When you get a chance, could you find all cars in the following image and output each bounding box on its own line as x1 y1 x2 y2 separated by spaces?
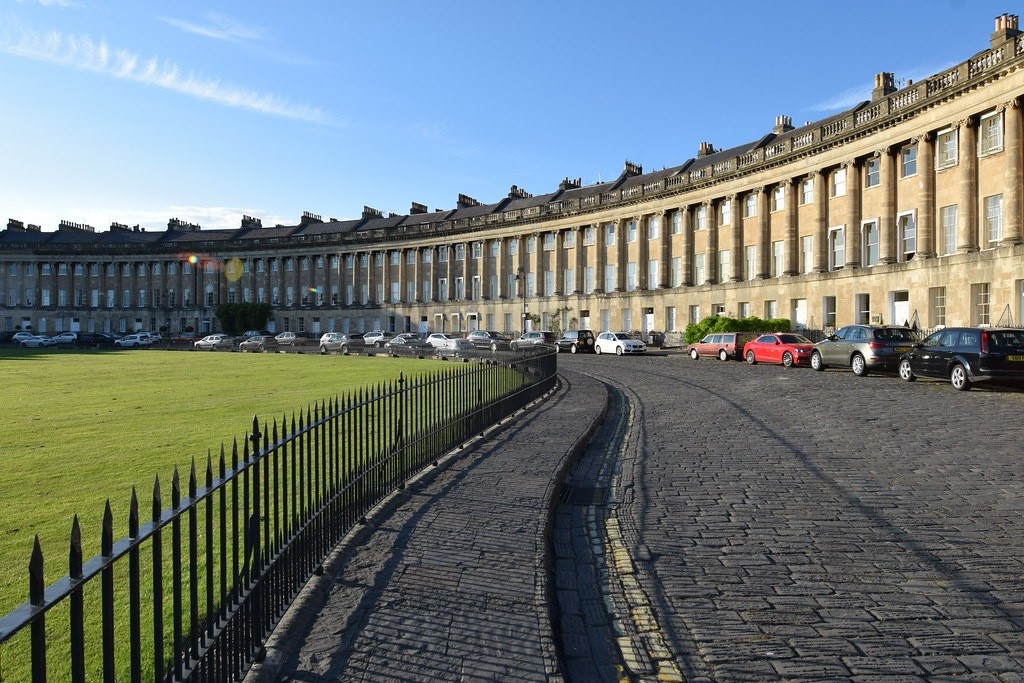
171 333 200 344
319 331 341 344
436 341 478 358
12 332 33 343
239 336 280 353
594 332 647 356
743 333 816 366
20 335 51 347
390 336 434 358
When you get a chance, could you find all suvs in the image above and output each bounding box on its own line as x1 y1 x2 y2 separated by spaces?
235 330 273 345
52 332 77 345
810 323 918 378
114 334 150 348
553 329 595 354
194 334 234 351
426 334 471 349
509 332 556 351
138 332 162 343
384 332 422 349
319 334 365 354
467 331 510 351
274 332 306 346
362 330 393 348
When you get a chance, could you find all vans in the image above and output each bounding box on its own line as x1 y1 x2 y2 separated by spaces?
687 333 758 361
79 332 114 346
897 326 1024 392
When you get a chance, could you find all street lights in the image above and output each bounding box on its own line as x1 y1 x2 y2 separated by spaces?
514 267 526 334
